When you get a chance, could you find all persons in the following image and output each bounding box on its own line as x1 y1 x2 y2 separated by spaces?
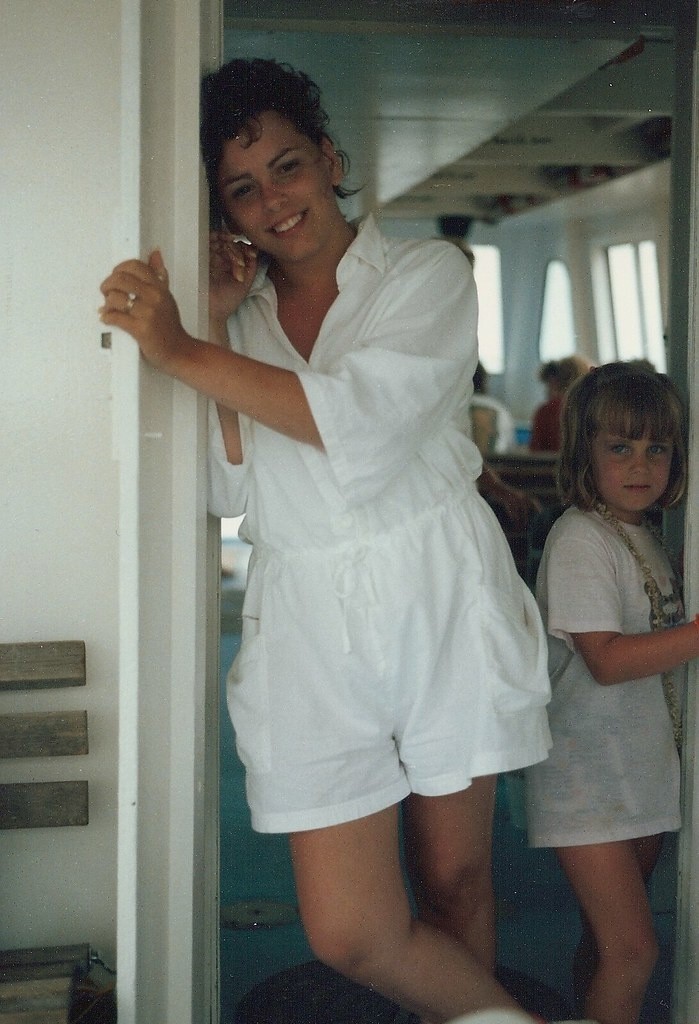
97 59 555 1024
527 360 699 1024
471 353 592 529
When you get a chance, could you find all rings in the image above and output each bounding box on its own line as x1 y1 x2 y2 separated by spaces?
123 293 137 314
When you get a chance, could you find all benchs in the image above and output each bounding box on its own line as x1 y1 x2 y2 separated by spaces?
1 641 89 1023
481 454 664 548
